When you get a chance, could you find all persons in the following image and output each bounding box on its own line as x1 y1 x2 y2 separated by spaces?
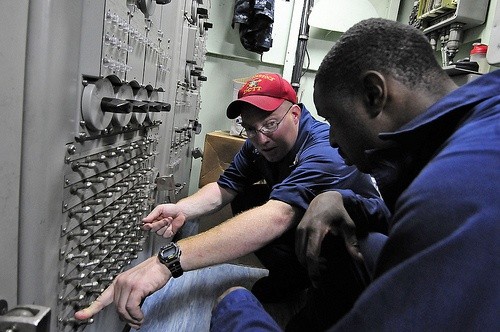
76 71 380 332
208 17 500 332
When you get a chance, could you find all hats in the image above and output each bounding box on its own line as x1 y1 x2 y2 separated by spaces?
226 72 298 119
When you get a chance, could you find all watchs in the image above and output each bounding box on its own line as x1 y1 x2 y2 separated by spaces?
157 241 184 279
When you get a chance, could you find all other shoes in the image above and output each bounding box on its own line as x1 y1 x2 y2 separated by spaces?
285 298 355 332
251 277 310 303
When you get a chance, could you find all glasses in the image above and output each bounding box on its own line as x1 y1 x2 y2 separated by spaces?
238 105 292 139
